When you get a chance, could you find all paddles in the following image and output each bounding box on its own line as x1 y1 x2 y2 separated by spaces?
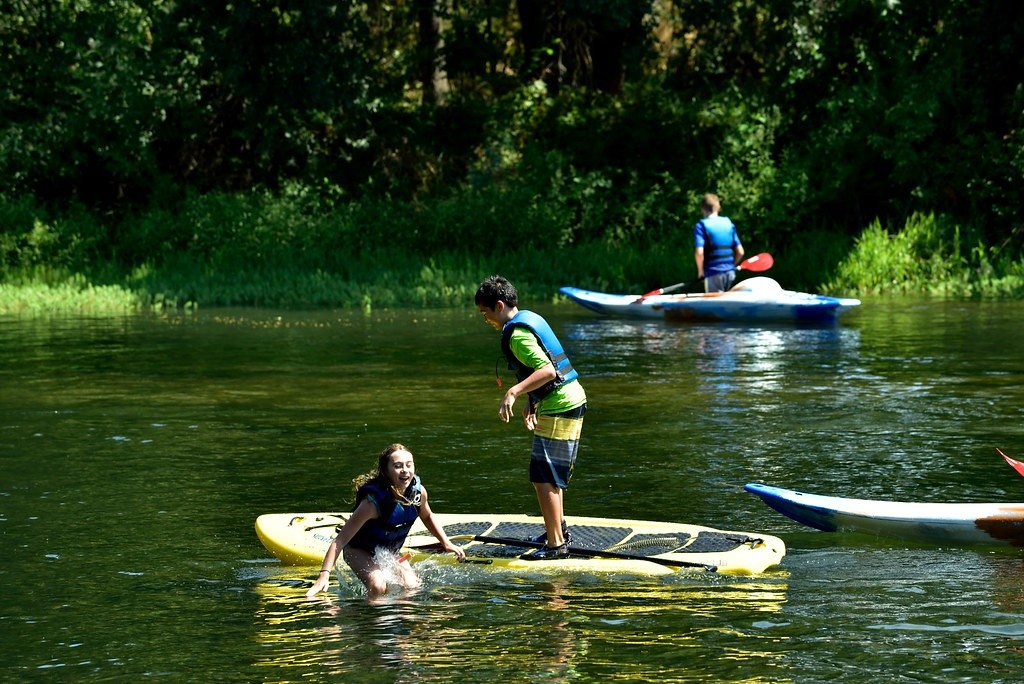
638 252 773 300
402 534 717 573
995 447 1024 477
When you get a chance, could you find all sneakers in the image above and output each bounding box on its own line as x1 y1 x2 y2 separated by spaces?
522 545 572 561
522 530 571 544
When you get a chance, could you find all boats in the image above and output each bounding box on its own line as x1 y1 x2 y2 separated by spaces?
560 276 863 322
744 480 1024 547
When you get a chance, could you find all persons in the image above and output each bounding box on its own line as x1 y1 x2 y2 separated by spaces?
693 192 744 292
306 444 464 597
474 275 588 559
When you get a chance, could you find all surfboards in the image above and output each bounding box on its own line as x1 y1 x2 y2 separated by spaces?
251 508 787 581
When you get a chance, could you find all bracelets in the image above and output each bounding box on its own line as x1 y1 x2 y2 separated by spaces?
320 570 329 573
